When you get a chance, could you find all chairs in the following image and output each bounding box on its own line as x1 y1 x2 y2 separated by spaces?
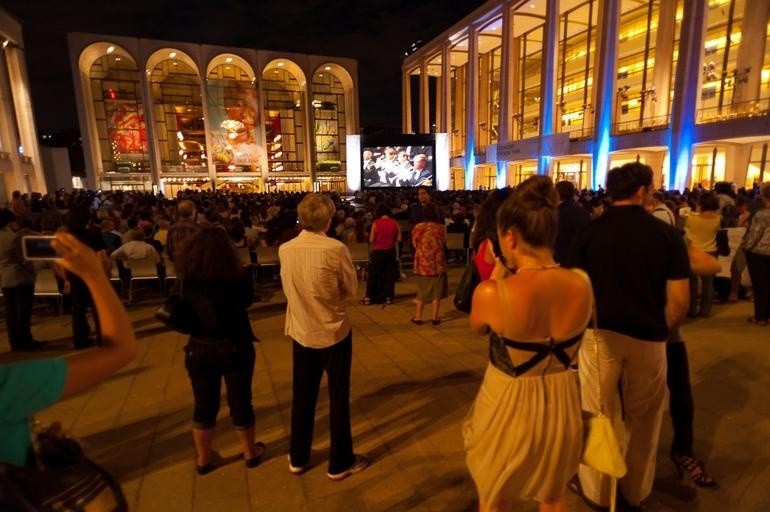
346 242 370 267
33 269 67 327
127 258 162 305
253 246 279 288
103 257 124 300
445 232 466 250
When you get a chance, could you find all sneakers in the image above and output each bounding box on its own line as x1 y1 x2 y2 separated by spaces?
327 454 368 479
196 450 215 475
287 452 304 474
246 441 265 468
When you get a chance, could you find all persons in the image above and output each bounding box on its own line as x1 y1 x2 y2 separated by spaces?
741 183 770 327
0 208 44 353
205 188 299 228
114 188 166 238
676 182 753 210
164 228 266 476
683 193 720 319
722 185 769 304
553 180 591 262
567 169 690 512
278 193 370 481
620 163 715 487
177 189 205 200
30 187 70 233
359 204 401 306
362 146 434 186
70 207 112 350
648 191 675 227
410 203 448 325
70 188 114 228
461 175 595 512
0 233 139 512
574 184 607 211
166 200 206 281
111 229 160 277
332 193 370 245
409 186 440 228
12 191 26 213
471 186 513 281
440 186 489 233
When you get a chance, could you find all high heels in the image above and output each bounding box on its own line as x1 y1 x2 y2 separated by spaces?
670 454 715 487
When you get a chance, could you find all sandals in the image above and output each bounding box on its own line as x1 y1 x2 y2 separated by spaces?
359 297 371 305
386 298 391 304
411 317 422 325
433 317 440 325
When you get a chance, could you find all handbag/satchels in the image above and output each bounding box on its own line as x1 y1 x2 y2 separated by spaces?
0 441 127 512
155 286 191 337
453 263 479 313
573 266 628 479
715 221 731 256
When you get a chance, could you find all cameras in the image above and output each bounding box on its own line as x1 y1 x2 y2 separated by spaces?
19 235 63 261
